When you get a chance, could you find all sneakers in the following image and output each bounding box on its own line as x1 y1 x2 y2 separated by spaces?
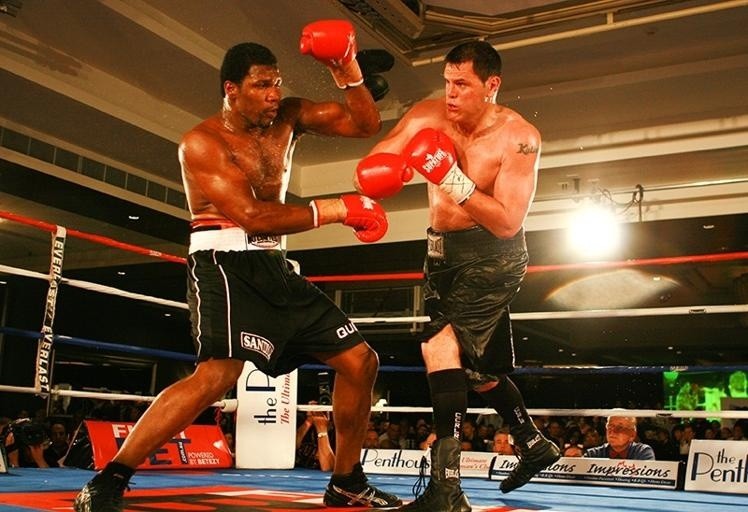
322 461 403 509
74 460 137 512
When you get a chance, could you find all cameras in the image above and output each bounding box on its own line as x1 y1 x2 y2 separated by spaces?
318 372 332 405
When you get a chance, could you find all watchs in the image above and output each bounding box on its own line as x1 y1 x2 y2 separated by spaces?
317 432 327 438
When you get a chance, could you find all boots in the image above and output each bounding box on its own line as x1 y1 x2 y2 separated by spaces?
499 417 563 495
389 434 474 512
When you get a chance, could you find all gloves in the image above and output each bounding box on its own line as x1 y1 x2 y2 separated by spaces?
299 19 365 89
311 152 410 244
405 126 477 206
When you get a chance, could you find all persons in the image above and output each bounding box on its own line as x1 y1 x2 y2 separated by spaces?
354 41 561 512
1 388 152 470
219 416 235 458
534 406 748 460
76 17 405 512
362 402 513 455
676 378 705 410
296 375 336 472
